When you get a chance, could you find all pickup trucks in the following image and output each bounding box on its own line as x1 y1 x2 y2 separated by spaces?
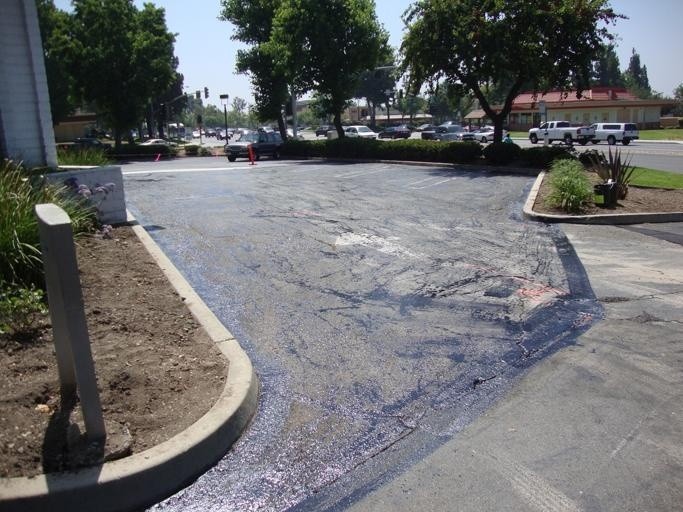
527 119 595 146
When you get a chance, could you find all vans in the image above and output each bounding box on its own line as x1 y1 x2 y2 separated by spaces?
589 122 638 145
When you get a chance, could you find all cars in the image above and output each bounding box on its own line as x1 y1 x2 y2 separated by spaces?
86 128 111 138
135 123 300 162
313 122 511 142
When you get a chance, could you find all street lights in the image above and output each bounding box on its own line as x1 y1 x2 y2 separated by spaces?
218 94 229 143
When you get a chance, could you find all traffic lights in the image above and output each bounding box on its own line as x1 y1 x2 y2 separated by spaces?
203 87 208 99
